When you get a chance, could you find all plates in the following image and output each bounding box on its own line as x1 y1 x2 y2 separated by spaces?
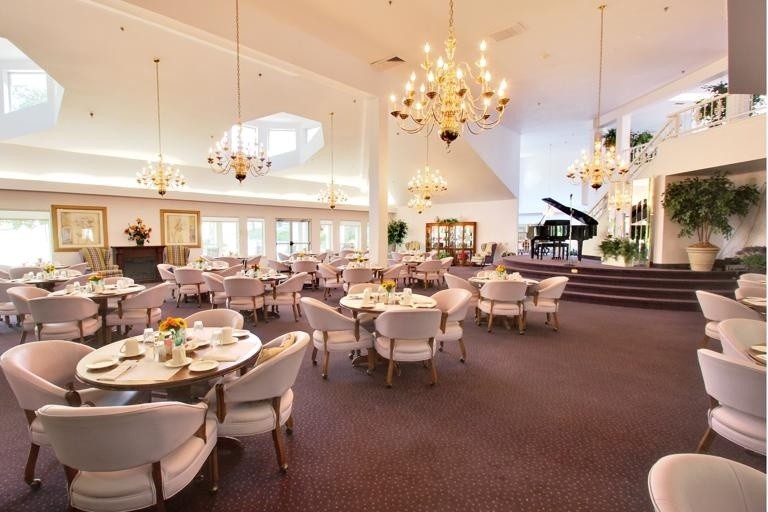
215 337 238 345
188 360 219 371
231 330 249 337
85 356 119 370
118 349 144 358
184 338 209 354
164 356 193 368
751 345 766 352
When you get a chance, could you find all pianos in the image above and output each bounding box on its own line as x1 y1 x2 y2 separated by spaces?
529 197 598 261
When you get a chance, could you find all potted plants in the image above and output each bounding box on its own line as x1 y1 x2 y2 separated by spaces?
655 164 763 274
596 231 643 270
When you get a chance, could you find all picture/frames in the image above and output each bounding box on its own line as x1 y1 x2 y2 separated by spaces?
158 207 202 249
49 203 110 253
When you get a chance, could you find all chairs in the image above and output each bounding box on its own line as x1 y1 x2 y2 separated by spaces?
632 262 768 510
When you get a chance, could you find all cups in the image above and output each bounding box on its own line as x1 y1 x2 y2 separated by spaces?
236 269 276 278
23 269 70 281
361 286 414 308
187 263 208 270
210 336 223 354
120 339 139 356
193 321 204 336
172 345 187 364
65 279 138 295
220 327 233 341
509 272 520 280
156 335 172 362
143 328 154 346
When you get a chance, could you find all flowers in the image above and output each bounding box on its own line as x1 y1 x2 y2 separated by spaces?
123 216 154 244
